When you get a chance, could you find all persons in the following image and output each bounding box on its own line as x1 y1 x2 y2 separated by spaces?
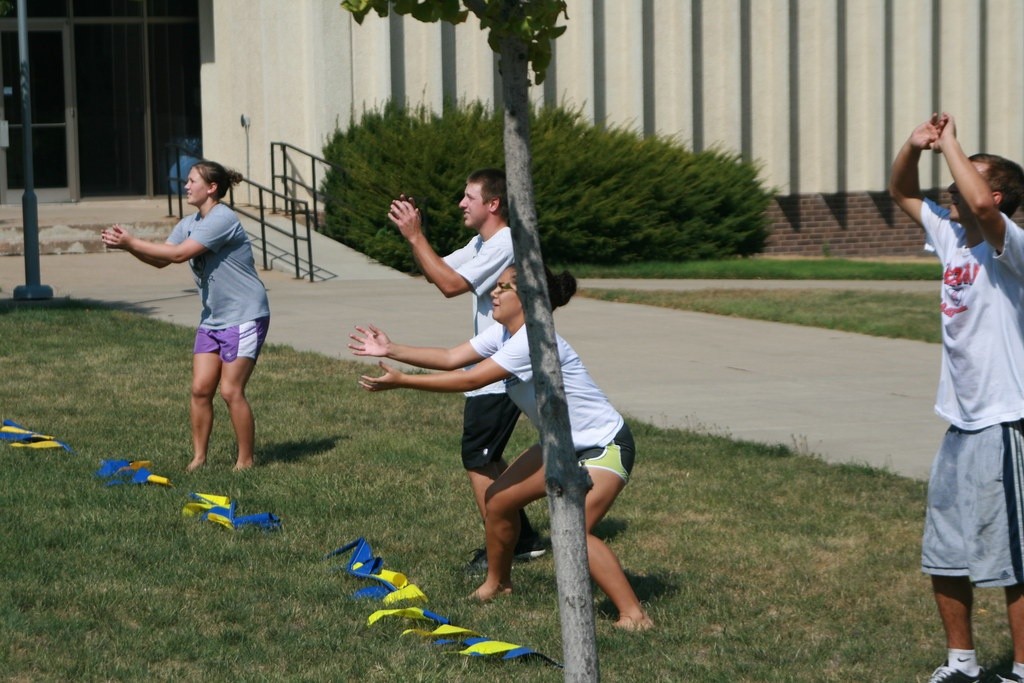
386 169 549 580
347 262 656 635
888 111 1023 683
103 163 273 477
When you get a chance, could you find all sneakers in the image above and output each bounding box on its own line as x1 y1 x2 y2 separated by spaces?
926 659 985 683
989 671 1024 683
513 533 546 558
470 547 488 576
